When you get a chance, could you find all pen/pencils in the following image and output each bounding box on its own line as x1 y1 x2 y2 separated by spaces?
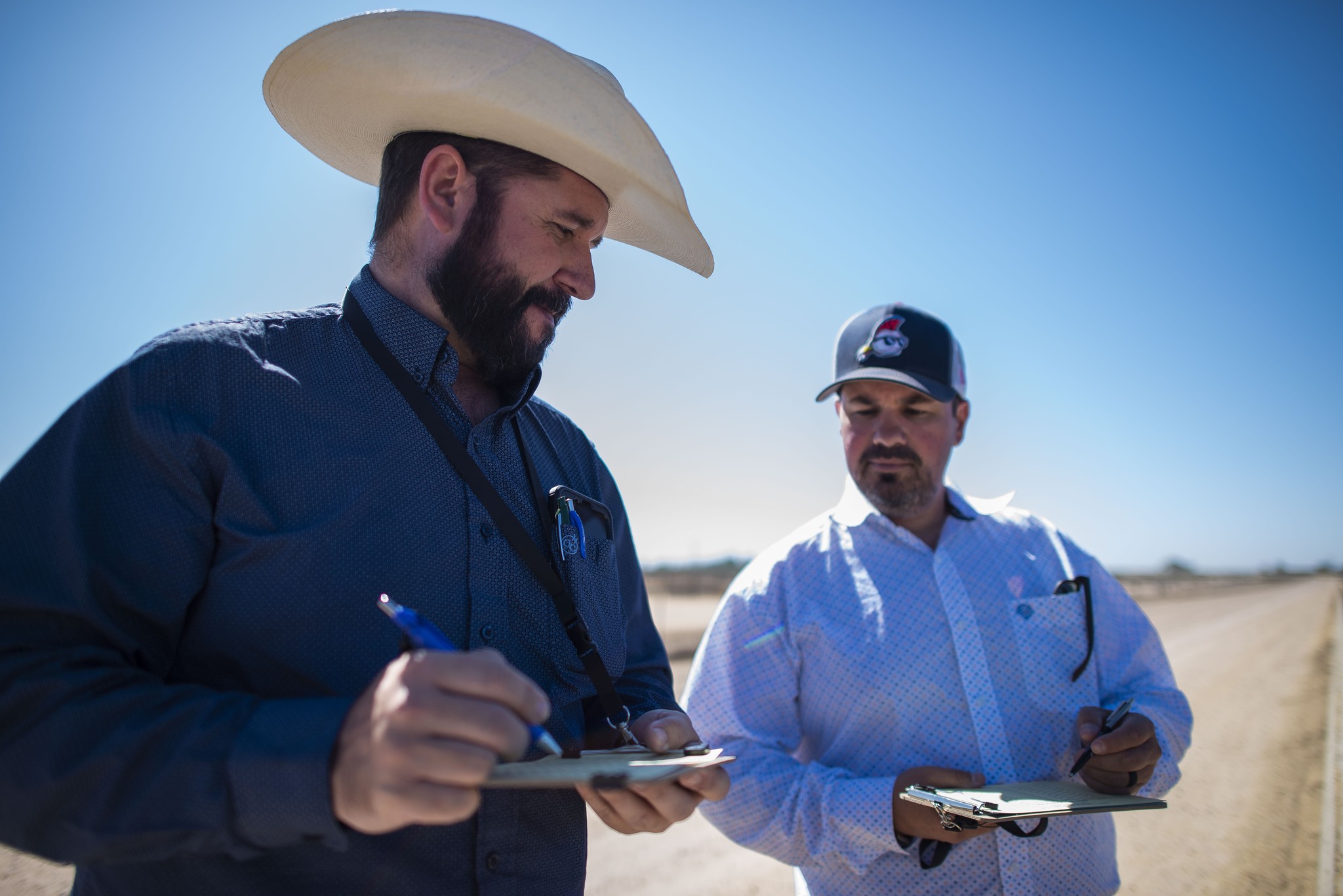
566 498 589 558
1068 695 1133 781
376 594 573 757
555 497 571 560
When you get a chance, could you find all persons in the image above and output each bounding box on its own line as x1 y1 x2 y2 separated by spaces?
676 301 1193 895
0 9 728 896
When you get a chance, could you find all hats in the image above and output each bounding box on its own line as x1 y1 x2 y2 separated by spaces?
261 10 719 282
816 303 970 408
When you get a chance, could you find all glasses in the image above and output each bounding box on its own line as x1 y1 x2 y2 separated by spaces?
1053 576 1095 681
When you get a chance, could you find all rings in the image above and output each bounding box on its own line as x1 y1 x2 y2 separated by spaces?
1126 771 1137 787
